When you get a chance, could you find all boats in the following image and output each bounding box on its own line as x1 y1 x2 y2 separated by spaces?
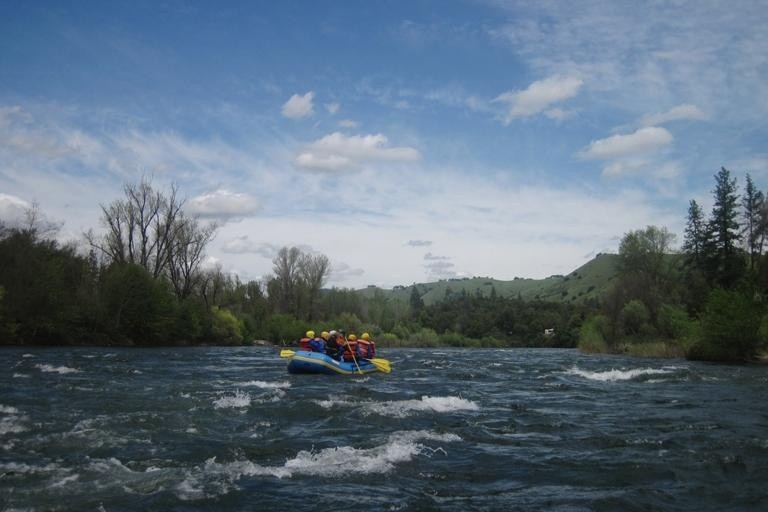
287 350 381 376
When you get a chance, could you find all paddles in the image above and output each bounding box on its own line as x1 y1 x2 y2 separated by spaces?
363 358 390 372
280 349 297 357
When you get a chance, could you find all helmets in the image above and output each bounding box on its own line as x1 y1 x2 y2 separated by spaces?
305 329 369 341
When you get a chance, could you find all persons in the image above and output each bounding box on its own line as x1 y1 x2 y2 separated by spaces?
300 329 376 362
